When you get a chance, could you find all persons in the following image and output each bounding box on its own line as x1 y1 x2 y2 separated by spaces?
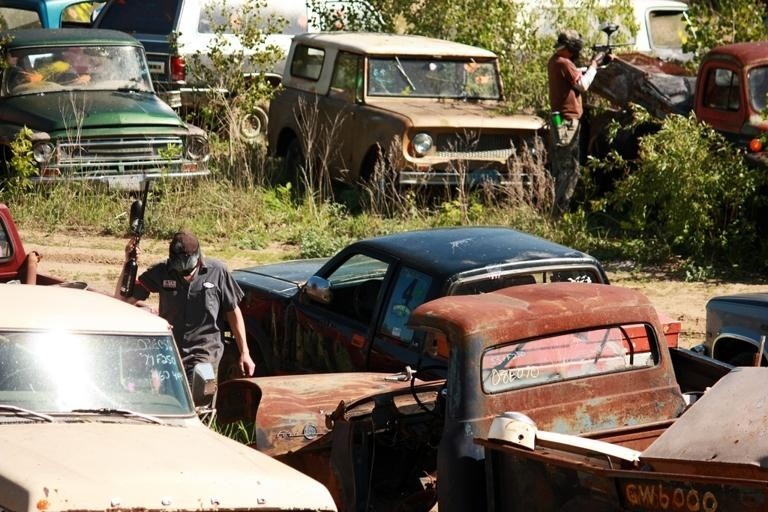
547 30 603 211
113 229 255 424
25 248 43 286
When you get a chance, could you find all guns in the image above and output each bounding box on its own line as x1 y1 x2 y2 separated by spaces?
120 181 149 296
589 25 634 65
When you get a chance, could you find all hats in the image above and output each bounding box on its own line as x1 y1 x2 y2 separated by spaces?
553 27 580 49
168 229 201 276
